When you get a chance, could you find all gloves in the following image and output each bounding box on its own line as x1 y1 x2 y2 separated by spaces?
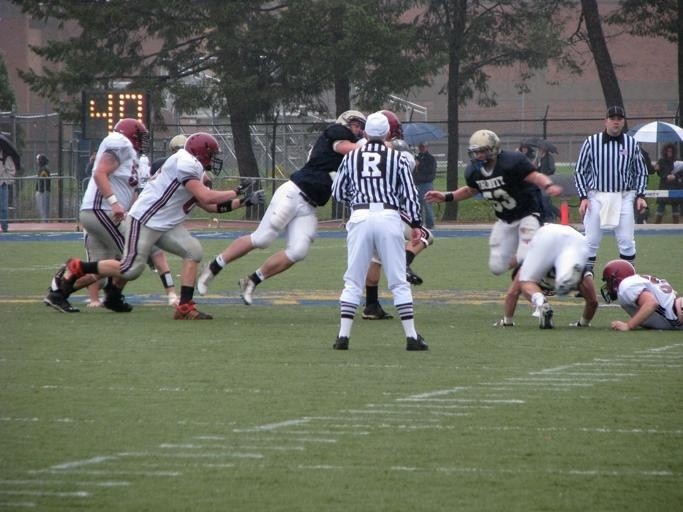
569 315 592 327
234 178 257 195
494 319 515 328
240 189 266 206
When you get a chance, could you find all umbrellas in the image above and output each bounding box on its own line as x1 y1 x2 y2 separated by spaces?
627 118 683 160
1 135 21 170
520 135 559 161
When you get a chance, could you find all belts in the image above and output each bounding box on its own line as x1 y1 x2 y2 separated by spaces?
299 192 318 208
353 202 398 211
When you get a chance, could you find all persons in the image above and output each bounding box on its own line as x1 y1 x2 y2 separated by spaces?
635 141 658 223
1 147 15 233
494 223 598 328
602 259 682 330
656 144 683 223
196 109 435 351
43 118 267 319
34 154 50 223
573 106 650 298
516 139 535 159
423 129 563 280
537 149 560 224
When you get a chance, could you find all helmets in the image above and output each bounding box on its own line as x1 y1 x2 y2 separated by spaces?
539 267 556 295
336 110 399 136
113 118 152 155
36 154 49 162
467 129 502 169
601 260 635 304
170 132 223 177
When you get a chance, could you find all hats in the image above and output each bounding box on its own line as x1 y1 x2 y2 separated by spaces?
607 107 626 118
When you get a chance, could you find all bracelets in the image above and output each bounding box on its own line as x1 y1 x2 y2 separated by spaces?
445 191 454 202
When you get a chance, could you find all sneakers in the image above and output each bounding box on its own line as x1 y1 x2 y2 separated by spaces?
406 264 423 285
406 333 428 350
102 277 133 312
539 303 554 328
333 336 350 350
45 290 80 313
174 300 213 319
362 302 395 320
239 275 256 306
52 259 85 292
197 261 215 295
